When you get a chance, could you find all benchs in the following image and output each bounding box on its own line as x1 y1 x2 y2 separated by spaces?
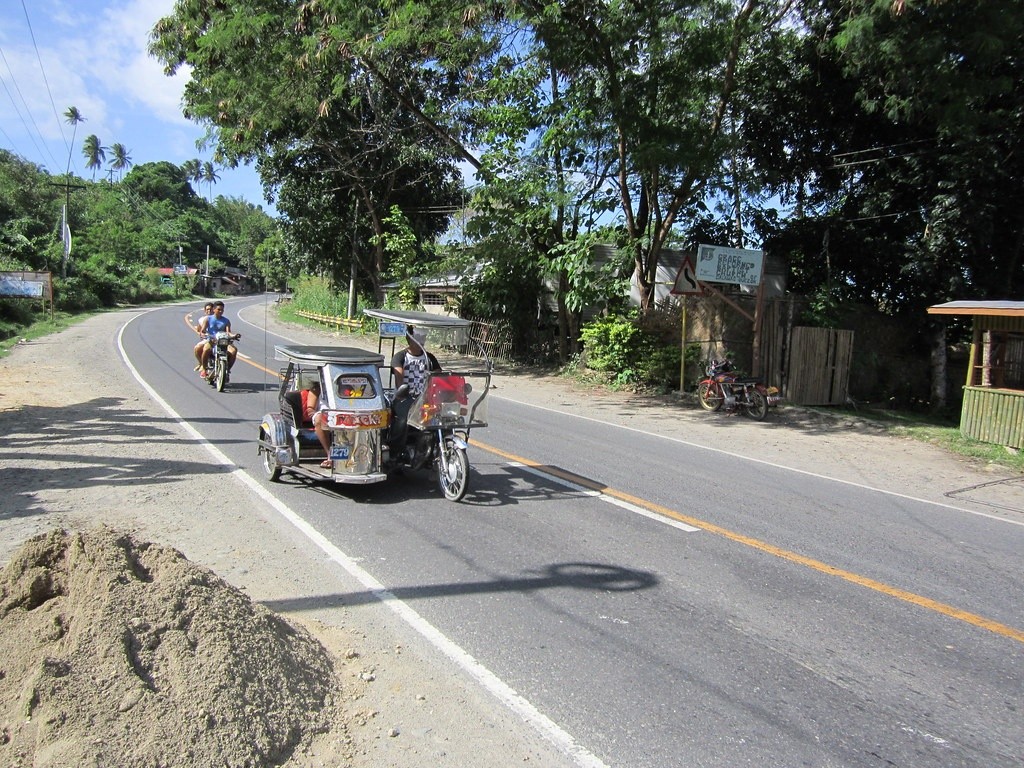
286 392 322 447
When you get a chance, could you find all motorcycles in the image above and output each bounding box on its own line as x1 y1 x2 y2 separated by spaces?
201 328 241 393
697 361 780 422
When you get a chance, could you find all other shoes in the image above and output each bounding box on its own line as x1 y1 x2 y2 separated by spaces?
194 365 201 371
321 461 331 468
200 371 206 378
388 458 403 465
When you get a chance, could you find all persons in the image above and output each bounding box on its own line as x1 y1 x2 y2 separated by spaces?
307 381 338 468
389 325 446 466
194 301 241 384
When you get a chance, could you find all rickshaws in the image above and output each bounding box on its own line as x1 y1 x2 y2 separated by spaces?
257 308 499 504
